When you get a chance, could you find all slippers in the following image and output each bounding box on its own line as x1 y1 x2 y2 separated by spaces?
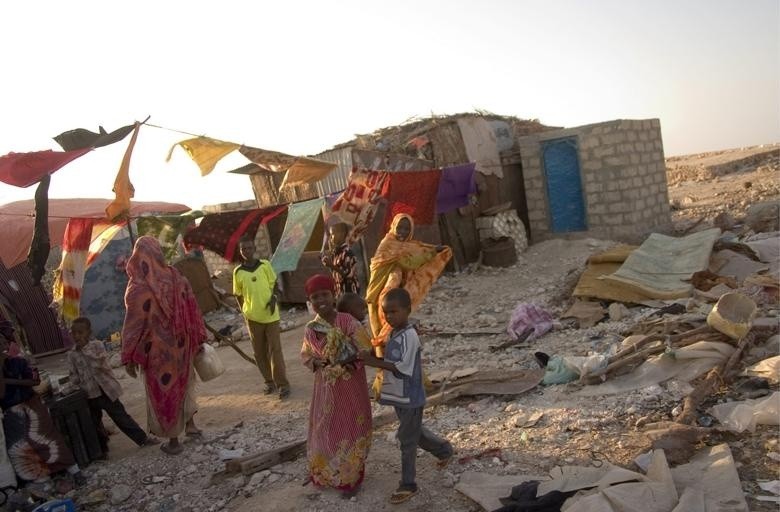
390 485 420 504
436 457 452 472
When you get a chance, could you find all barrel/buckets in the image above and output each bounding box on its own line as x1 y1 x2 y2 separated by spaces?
195 342 224 382
110 332 121 342
479 237 518 266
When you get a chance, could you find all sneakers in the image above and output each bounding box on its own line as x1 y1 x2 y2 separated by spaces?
185 428 202 437
145 435 161 445
72 471 98 490
160 442 183 454
278 387 290 399
264 385 275 396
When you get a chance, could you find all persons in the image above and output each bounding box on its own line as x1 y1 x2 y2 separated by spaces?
355 288 453 505
0 320 26 357
232 236 291 400
0 333 82 496
364 213 454 400
299 272 373 498
67 317 163 453
119 236 208 455
321 222 360 302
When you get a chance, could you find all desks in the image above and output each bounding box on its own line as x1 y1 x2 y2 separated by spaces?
42 387 103 466
